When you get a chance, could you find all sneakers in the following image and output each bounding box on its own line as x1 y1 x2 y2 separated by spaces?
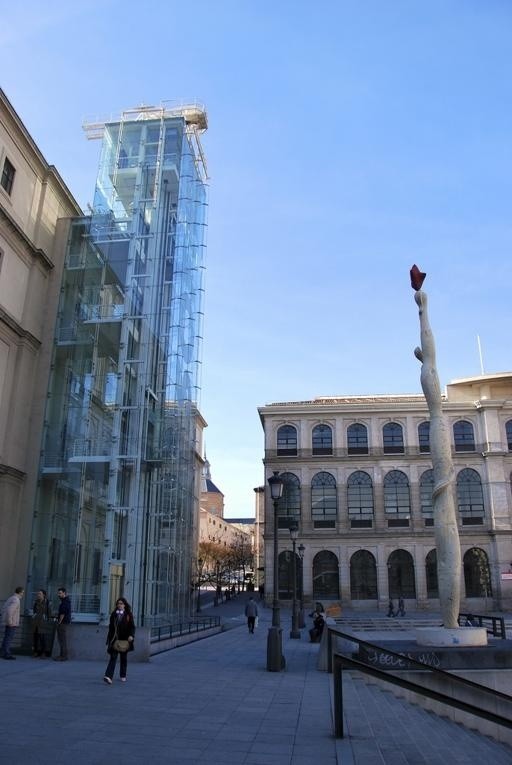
104 677 112 684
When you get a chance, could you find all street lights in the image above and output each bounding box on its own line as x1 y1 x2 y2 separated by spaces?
267 471 287 672
227 559 231 600
297 544 305 628
215 560 221 606
196 557 204 613
288 520 301 639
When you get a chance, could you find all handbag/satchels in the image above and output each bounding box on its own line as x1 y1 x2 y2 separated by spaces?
114 640 129 652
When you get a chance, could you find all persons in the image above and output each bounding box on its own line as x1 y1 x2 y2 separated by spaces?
31 590 50 658
224 589 229 601
232 585 235 599
309 602 324 643
386 596 409 619
245 596 259 634
1 587 25 660
104 598 135 684
54 588 72 661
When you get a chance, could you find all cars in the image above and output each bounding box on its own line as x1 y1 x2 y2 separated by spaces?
200 575 209 582
207 571 215 577
211 566 244 585
